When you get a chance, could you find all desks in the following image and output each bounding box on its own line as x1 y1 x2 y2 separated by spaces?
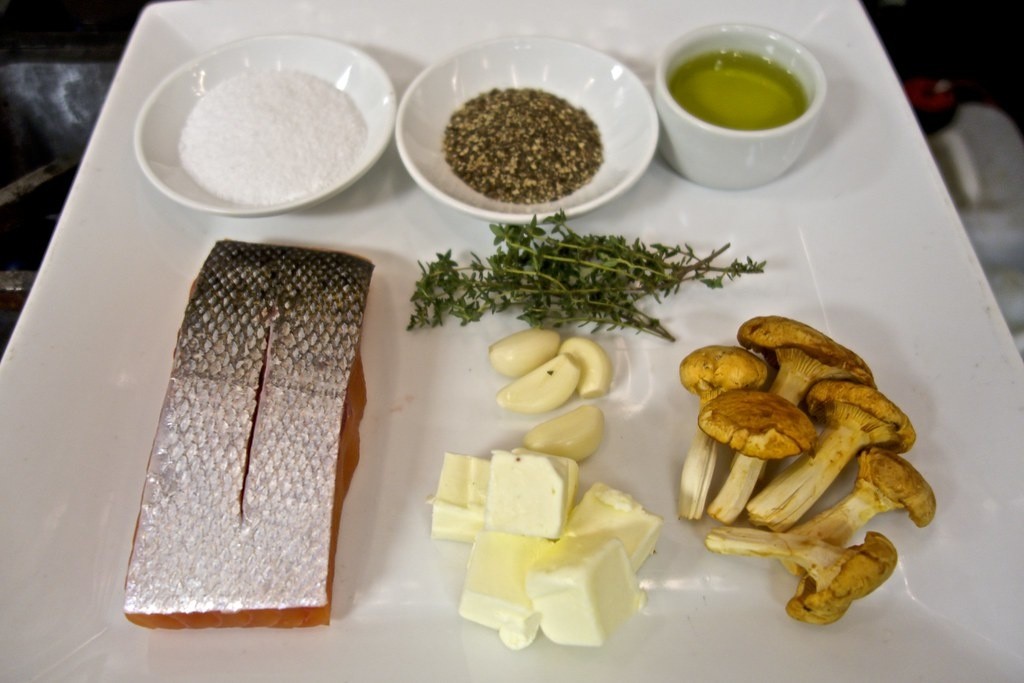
0 0 1024 683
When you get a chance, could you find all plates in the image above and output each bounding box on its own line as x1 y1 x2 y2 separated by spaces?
395 36 661 224
0 0 1024 683
133 32 398 218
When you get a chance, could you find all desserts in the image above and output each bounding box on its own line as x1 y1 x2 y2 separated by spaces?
426 445 662 648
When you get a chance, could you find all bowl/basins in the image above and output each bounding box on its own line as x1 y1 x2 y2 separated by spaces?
651 23 829 194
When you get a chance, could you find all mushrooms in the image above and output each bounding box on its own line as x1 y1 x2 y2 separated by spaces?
677 315 936 625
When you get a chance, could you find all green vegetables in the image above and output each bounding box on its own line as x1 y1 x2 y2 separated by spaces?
405 208 767 342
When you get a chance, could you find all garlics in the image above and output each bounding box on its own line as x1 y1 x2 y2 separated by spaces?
487 328 611 463
